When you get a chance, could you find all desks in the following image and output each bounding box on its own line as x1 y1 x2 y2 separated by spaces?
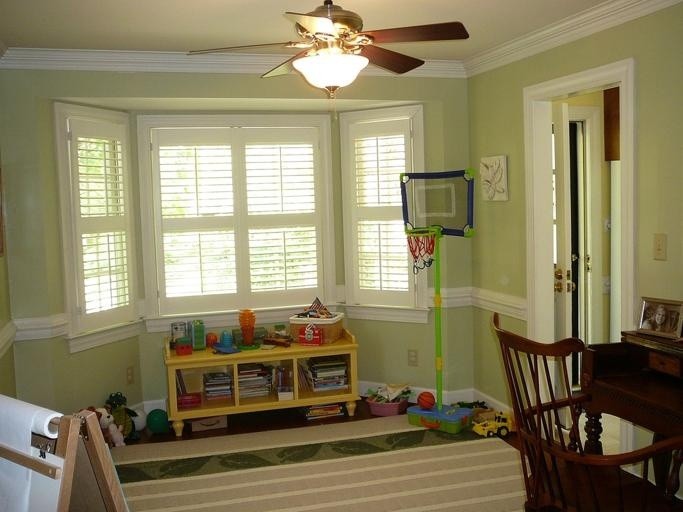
581 326 682 493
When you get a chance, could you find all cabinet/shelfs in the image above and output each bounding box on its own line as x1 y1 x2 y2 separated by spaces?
160 330 361 437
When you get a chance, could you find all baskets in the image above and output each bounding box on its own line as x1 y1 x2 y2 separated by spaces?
366 395 408 416
289 312 344 344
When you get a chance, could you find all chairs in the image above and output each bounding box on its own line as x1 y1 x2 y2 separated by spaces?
492 312 682 512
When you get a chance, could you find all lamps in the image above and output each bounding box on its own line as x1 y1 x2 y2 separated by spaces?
292 49 368 96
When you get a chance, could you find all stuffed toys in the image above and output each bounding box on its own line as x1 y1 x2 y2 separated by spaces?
88 392 172 446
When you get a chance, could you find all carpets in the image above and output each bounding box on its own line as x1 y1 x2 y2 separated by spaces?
109 412 534 512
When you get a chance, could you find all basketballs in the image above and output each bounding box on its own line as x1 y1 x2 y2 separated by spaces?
417 392 435 409
206 333 217 346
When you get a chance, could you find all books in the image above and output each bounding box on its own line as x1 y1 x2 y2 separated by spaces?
173 356 350 420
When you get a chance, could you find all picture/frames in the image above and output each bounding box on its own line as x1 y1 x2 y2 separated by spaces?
635 296 682 340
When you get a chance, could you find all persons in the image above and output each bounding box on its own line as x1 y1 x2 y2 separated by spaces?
640 303 671 330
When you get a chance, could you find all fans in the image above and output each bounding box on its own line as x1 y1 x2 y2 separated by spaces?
187 0 468 79
